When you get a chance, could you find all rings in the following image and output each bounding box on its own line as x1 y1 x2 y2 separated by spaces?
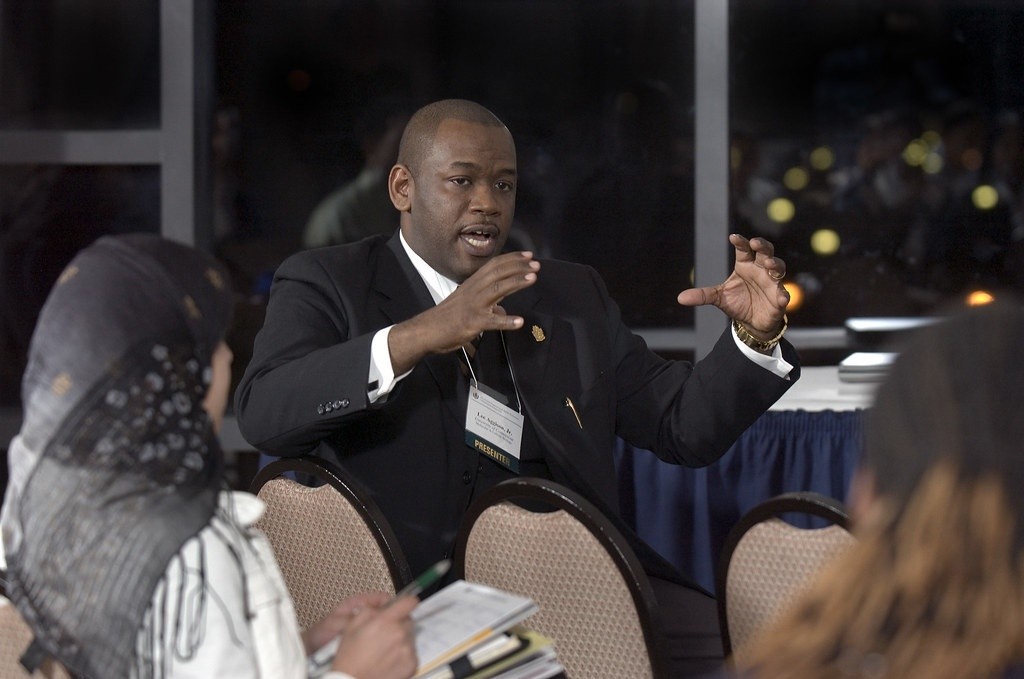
768 270 786 280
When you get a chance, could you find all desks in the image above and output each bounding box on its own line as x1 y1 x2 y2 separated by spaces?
603 367 888 595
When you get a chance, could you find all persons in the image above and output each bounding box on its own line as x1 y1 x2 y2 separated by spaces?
726 300 1024 679
0 235 421 679
235 100 801 679
301 114 418 251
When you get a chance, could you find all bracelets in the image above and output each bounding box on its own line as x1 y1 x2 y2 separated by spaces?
733 313 789 351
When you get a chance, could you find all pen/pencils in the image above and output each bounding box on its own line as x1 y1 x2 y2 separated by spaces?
377 558 452 613
562 396 583 431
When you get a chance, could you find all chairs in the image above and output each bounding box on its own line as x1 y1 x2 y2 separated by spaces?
452 478 667 679
718 492 859 679
249 454 413 633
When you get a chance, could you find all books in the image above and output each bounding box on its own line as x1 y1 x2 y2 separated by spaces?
415 625 565 679
